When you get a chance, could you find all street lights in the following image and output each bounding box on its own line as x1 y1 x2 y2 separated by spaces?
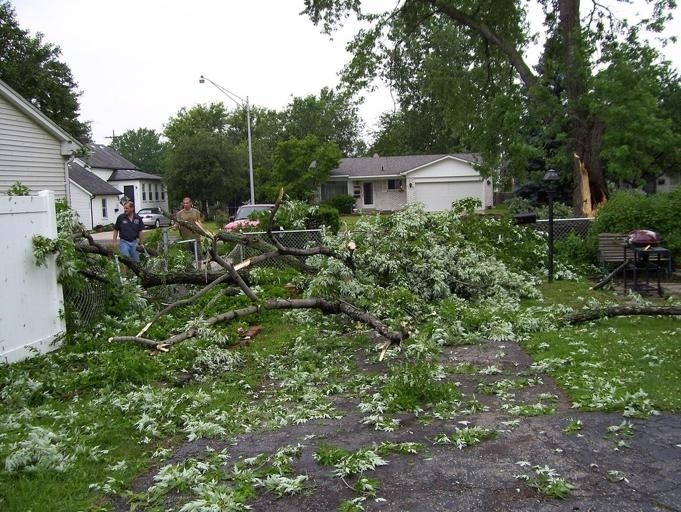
542 166 564 284
198 73 257 204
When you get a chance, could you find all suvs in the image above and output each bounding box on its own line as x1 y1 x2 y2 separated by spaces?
223 201 282 242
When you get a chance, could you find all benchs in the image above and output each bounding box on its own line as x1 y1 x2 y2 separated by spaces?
596 231 670 281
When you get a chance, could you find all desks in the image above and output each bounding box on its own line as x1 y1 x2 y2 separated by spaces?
614 238 669 300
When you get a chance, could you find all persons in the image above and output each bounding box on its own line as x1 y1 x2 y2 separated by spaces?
170 197 202 267
111 201 145 276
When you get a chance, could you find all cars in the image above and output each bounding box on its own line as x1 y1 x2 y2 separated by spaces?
136 206 176 228
